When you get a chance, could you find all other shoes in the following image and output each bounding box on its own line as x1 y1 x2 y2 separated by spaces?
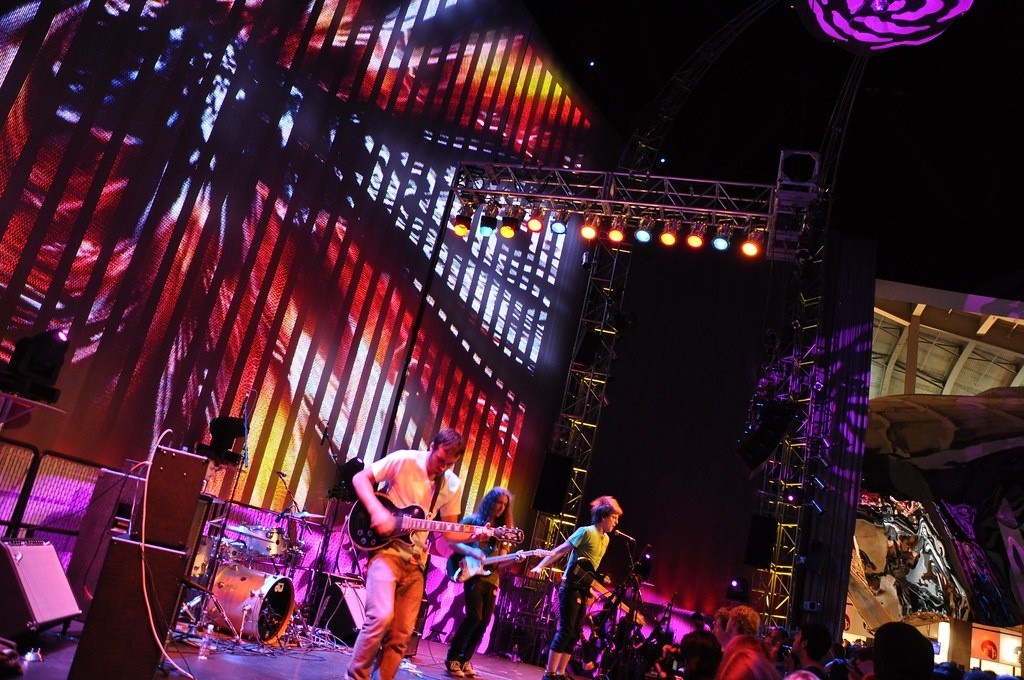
447 661 464 676
541 674 567 680
461 661 476 675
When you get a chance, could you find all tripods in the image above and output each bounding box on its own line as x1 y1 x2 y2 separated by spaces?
178 403 248 647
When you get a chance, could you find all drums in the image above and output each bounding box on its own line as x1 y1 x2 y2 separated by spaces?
246 526 286 558
190 535 215 578
201 561 295 645
210 537 248 562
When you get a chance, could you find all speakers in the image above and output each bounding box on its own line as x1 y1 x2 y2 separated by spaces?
530 452 577 514
804 539 832 575
737 399 798 471
67 468 145 621
129 445 210 548
309 581 369 644
68 536 188 680
0 537 82 645
743 512 778 573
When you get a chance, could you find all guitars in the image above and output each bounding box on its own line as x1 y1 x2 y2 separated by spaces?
566 557 647 627
446 546 556 583
348 492 525 551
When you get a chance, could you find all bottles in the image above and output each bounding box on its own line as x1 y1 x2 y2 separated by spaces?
198 624 214 659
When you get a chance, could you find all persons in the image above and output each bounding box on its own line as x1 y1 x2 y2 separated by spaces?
444 487 526 677
344 430 494 680
656 606 1024 680
530 496 622 680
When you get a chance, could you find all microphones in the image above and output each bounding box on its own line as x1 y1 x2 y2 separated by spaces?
275 509 287 523
321 422 329 445
274 470 286 477
239 393 249 417
615 530 635 543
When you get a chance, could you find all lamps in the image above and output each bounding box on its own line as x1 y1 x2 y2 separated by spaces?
501 205 526 238
550 210 571 234
526 208 546 233
684 221 707 249
739 231 765 256
607 216 627 242
634 215 656 243
479 202 499 237
713 224 733 250
581 214 601 239
451 197 473 237
659 218 682 246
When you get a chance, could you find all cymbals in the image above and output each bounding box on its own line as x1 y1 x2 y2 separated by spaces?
284 512 327 518
207 522 275 543
201 492 225 503
